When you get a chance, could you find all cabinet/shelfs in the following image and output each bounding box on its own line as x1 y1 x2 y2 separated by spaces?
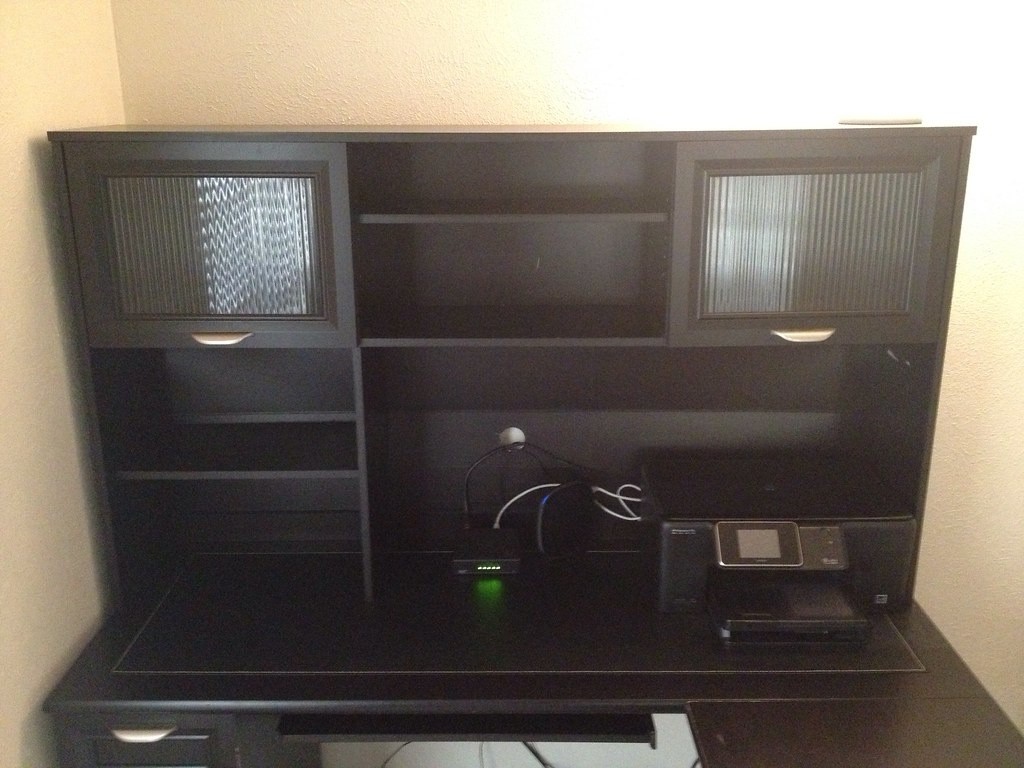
41 119 1022 767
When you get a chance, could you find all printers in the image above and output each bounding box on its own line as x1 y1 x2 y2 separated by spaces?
638 446 917 643
